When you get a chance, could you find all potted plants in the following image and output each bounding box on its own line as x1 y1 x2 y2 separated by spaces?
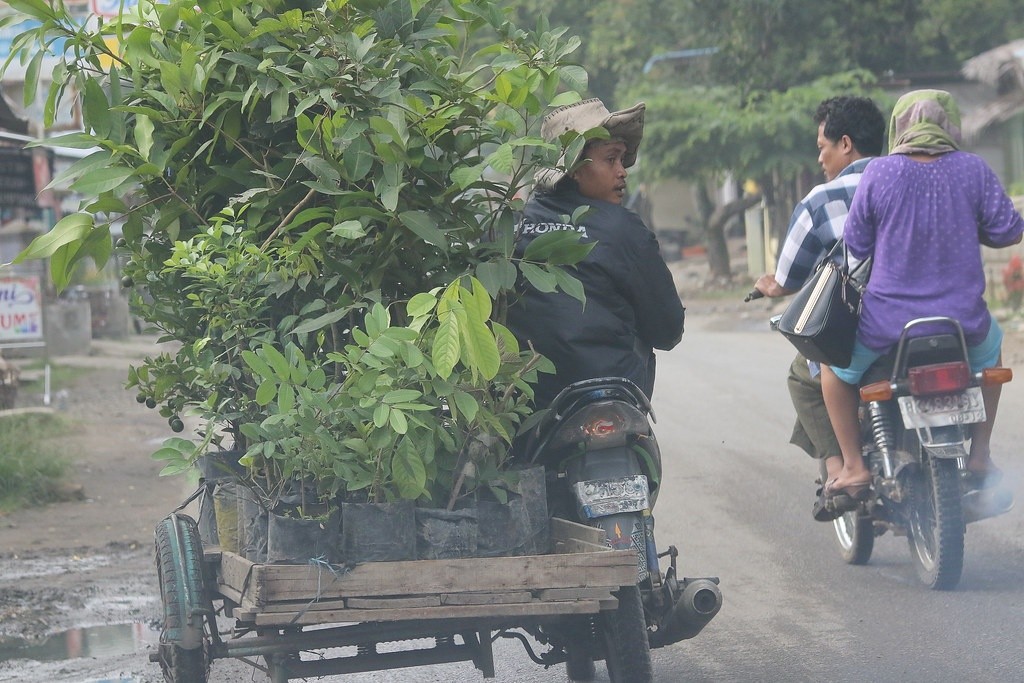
0 1 615 562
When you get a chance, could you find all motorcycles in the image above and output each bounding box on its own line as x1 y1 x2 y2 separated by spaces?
742 269 1017 593
150 352 724 683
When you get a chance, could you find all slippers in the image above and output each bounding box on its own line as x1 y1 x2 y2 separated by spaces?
968 468 1004 489
824 477 872 496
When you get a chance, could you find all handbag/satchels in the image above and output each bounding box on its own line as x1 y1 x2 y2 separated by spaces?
778 235 874 369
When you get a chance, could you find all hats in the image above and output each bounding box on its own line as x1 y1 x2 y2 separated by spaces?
533 96 646 194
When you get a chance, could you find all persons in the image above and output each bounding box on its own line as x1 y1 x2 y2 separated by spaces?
754 95 886 522
823 89 1024 494
471 95 685 461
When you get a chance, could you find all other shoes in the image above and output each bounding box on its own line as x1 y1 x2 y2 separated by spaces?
812 486 845 522
